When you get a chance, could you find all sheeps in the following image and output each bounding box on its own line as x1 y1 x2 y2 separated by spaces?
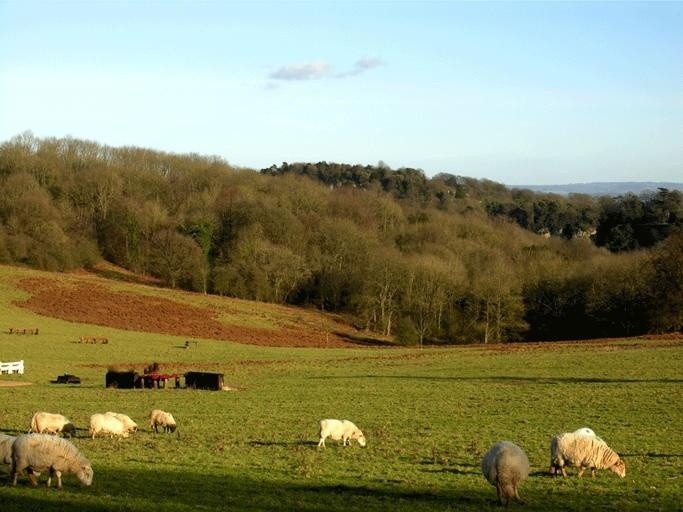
315 418 367 449
481 441 530 505
89 411 138 440
550 426 627 481
28 411 77 438
0 432 93 489
149 409 177 435
